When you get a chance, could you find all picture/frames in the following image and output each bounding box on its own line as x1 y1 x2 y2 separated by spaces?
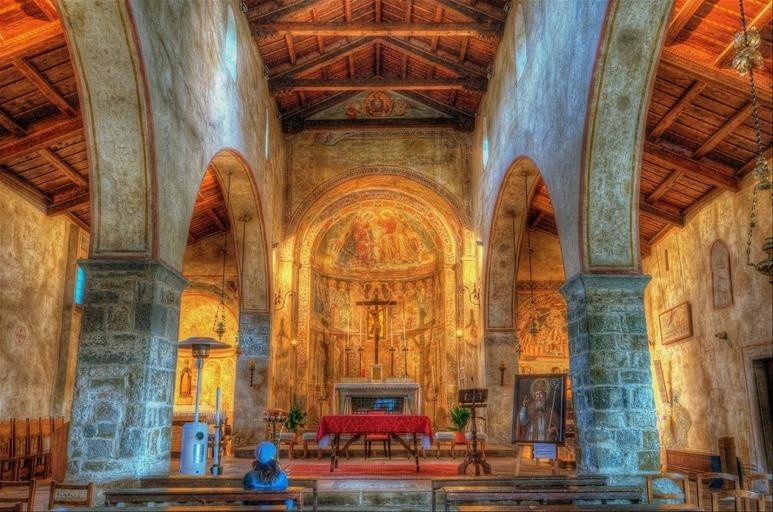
511 373 566 443
371 364 383 384
659 300 693 344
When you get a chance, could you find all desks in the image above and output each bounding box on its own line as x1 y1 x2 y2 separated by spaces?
317 414 431 472
431 475 700 511
435 431 488 459
50 473 319 512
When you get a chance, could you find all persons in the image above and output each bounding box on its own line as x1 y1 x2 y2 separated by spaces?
334 210 428 266
367 304 384 334
516 376 559 441
243 441 295 511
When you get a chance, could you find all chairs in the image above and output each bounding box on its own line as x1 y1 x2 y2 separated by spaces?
262 408 334 459
0 478 94 512
365 410 392 458
644 470 773 512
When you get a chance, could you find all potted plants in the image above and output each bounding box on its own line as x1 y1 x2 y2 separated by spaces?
283 400 308 445
448 406 471 442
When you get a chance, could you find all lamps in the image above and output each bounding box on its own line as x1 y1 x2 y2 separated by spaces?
520 169 542 334
174 336 232 474
247 359 258 387
728 0 773 284
212 217 232 339
